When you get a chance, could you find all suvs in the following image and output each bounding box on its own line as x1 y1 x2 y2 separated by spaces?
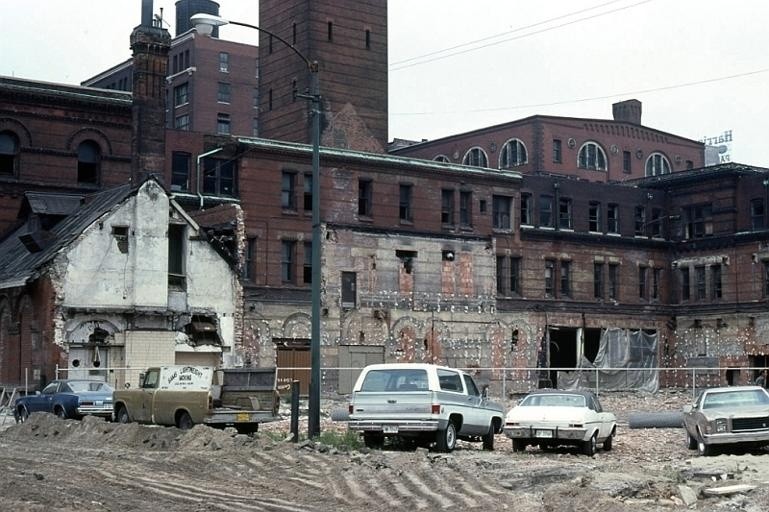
346 361 506 451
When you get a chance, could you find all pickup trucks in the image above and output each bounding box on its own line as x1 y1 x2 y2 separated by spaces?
111 363 282 434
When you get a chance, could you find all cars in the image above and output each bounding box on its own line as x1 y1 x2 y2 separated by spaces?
681 384 769 455
502 388 618 458
13 377 117 423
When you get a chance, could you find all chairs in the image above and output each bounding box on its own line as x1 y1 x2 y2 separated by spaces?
399 384 418 391
440 382 457 391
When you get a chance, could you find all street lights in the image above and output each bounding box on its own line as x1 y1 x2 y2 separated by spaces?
188 12 325 439
